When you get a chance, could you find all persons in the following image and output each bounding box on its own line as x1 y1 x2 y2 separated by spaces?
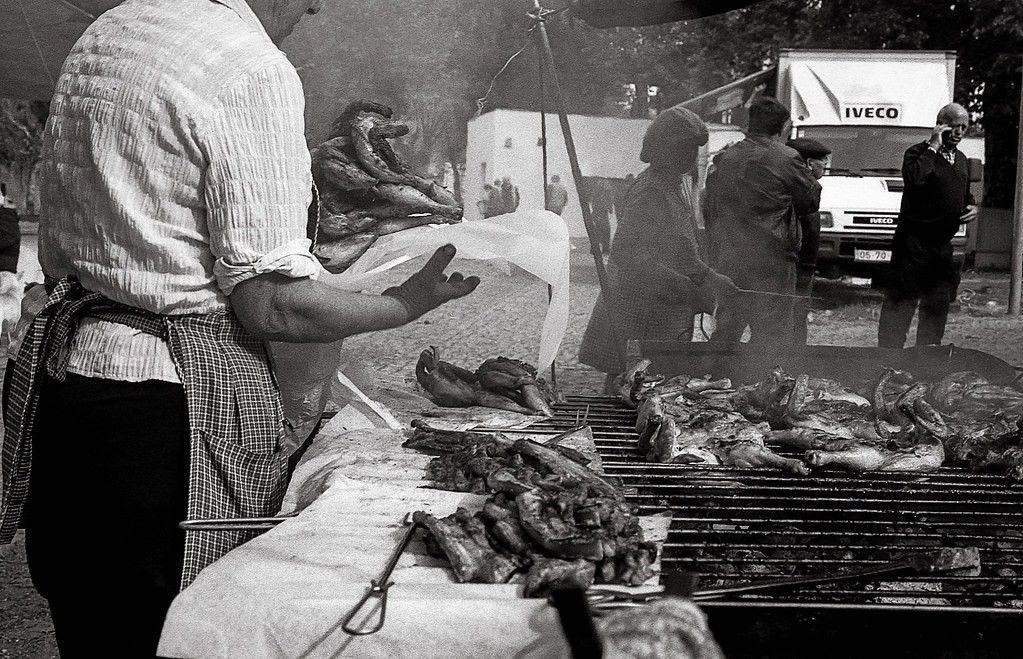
0 0 480 658
476 178 520 219
546 175 568 216
0 181 22 272
590 174 639 254
878 102 980 351
577 106 740 396
699 97 833 346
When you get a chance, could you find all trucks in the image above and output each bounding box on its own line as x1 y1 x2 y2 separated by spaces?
660 51 983 267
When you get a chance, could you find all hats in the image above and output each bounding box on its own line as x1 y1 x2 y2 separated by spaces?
640 106 709 162
786 138 831 157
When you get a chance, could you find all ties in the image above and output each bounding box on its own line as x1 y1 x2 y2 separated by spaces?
948 150 955 165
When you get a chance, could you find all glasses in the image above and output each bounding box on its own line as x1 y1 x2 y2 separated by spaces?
817 158 829 166
